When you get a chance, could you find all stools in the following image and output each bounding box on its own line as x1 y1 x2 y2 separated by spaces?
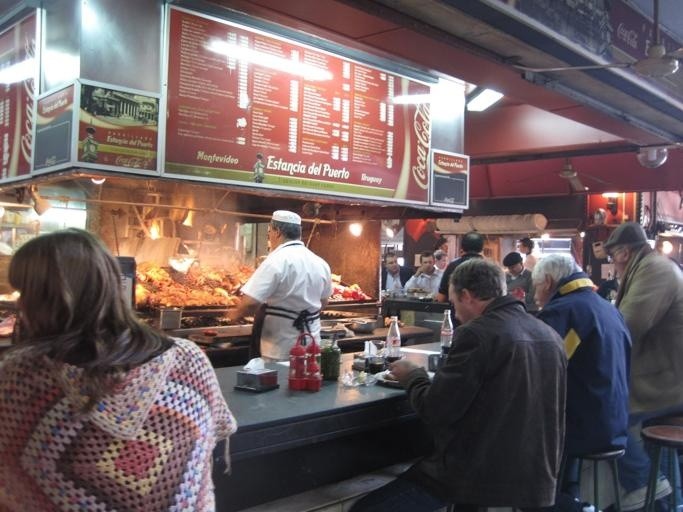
641 414 683 512
574 445 626 512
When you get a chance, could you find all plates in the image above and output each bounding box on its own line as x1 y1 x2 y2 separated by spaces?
374 370 436 385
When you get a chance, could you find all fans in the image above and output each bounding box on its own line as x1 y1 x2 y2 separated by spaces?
510 1 683 80
517 157 611 192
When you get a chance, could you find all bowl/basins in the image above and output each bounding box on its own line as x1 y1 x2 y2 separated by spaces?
349 318 377 333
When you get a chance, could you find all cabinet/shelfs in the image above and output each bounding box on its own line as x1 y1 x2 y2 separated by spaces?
0 202 40 256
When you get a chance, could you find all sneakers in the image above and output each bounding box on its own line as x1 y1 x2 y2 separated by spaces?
614 475 674 512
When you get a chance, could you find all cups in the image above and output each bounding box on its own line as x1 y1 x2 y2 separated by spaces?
428 354 449 371
367 340 386 366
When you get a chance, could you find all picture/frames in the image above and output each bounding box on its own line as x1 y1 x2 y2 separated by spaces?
32 80 73 176
73 77 163 177
159 3 434 206
0 7 41 185
431 149 470 209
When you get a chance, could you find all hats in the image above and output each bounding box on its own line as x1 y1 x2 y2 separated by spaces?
272 210 301 225
603 221 647 247
503 253 522 266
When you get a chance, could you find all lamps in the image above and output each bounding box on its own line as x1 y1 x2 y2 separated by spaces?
29 184 51 216
349 218 368 237
466 87 504 113
636 145 669 169
182 211 193 228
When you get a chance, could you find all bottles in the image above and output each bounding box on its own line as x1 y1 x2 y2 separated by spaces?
440 308 454 354
288 333 341 393
386 315 401 357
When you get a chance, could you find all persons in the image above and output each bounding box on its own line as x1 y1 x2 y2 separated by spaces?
602 220 683 511
351 259 568 508
224 208 333 362
380 231 540 311
581 224 619 299
0 227 239 512
533 252 633 512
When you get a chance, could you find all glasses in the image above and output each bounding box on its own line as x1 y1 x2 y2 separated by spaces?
608 247 625 258
